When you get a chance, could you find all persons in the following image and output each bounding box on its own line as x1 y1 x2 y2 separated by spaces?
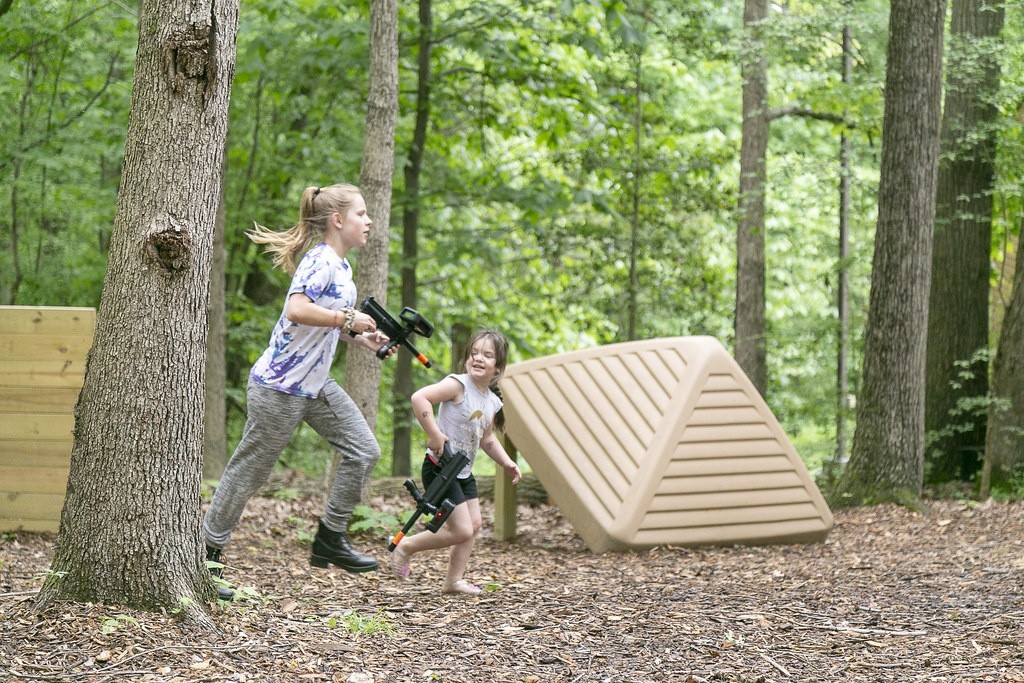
205 183 397 599
393 329 523 597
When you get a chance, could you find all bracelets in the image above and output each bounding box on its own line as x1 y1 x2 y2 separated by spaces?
332 308 338 329
340 305 356 335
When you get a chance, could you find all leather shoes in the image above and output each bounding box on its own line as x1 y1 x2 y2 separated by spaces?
202 544 234 601
308 518 380 570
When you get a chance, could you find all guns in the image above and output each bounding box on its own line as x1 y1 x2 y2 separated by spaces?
388 440 471 553
348 296 433 369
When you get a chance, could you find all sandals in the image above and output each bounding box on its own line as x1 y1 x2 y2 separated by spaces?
387 534 409 581
439 579 482 595
